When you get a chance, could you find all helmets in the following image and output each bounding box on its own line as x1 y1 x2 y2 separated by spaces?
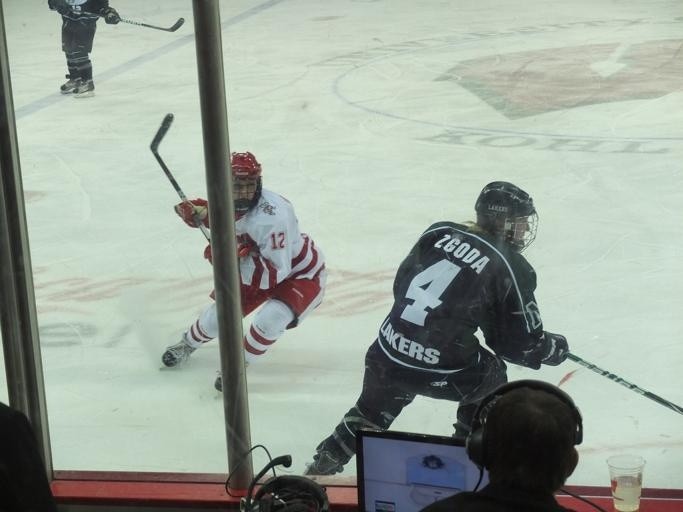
231 152 262 212
475 181 539 253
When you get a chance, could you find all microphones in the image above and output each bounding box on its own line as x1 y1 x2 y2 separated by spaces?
245 455 291 512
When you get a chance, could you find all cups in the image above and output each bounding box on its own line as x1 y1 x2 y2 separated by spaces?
605 452 648 512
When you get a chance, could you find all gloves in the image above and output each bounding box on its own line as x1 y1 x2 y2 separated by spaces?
175 199 208 227
104 8 120 24
543 332 568 366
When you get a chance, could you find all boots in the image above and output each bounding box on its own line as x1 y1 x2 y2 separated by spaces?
163 340 195 367
61 79 94 93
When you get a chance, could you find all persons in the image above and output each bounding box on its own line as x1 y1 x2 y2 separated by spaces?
307 179 570 479
419 385 580 512
48 0 121 95
159 149 329 391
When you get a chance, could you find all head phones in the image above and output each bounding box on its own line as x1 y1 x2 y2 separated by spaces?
466 379 582 472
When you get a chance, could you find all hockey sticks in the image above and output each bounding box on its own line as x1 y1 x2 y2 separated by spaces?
95 12 184 32
149 114 210 241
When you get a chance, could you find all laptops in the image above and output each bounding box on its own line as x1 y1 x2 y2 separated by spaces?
357 428 491 512
